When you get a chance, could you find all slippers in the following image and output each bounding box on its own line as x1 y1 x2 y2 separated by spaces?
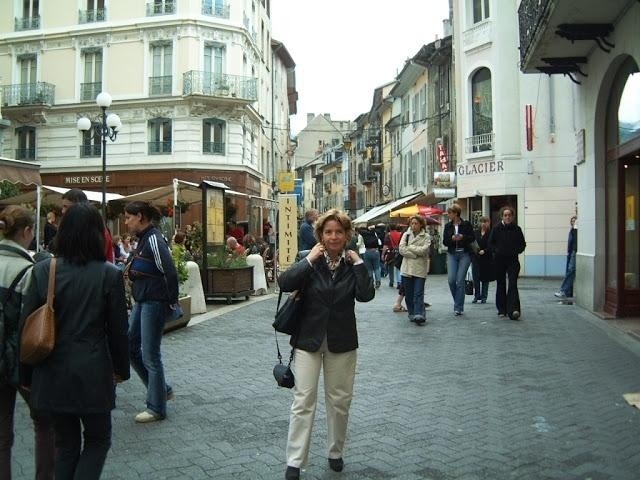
393 305 408 312
424 303 432 307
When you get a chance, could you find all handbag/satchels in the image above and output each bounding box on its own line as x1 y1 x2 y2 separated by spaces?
19 257 57 366
462 219 480 255
0 264 35 360
384 247 403 264
394 234 409 271
274 248 310 335
273 365 294 388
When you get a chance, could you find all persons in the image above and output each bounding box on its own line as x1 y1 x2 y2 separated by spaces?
130 236 138 251
32 236 56 263
172 232 186 262
119 232 133 259
19 202 131 478
299 204 526 323
554 216 578 297
105 227 113 263
44 212 58 249
112 235 127 265
277 208 375 480
184 225 194 255
2 202 35 477
26 236 36 257
226 220 276 258
124 200 179 422
61 189 88 215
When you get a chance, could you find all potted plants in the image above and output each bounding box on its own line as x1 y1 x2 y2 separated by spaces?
206 246 254 297
162 243 191 331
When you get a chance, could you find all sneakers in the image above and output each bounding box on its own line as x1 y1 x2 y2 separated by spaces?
554 291 565 297
166 389 174 400
413 315 426 325
511 310 520 320
135 408 165 422
285 466 300 480
328 458 343 472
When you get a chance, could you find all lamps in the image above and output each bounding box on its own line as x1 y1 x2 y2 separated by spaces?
343 133 354 152
335 162 342 174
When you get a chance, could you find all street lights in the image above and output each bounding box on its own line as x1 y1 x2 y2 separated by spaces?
77 92 123 227
